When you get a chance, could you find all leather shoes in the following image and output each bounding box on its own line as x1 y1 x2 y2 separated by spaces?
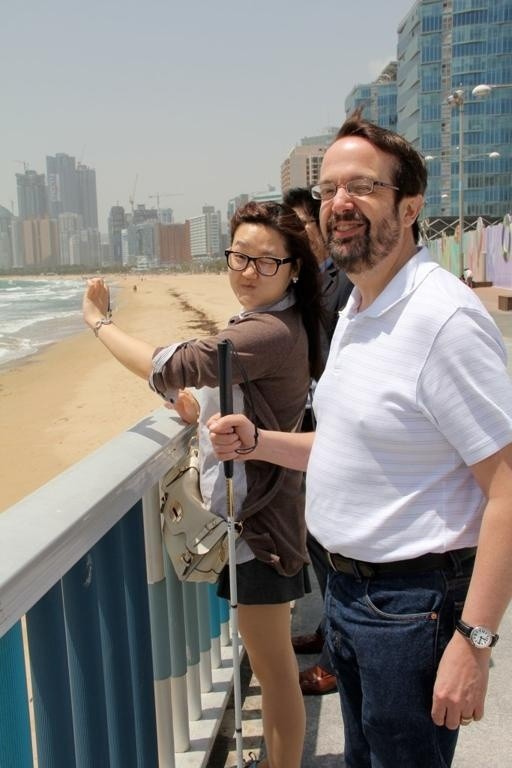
298 663 337 694
290 632 325 655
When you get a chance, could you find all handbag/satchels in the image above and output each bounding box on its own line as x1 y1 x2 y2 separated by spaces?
158 436 244 585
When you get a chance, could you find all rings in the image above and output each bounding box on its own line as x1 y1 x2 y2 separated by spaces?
460 718 474 724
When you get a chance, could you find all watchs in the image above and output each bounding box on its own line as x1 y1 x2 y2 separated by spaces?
455 619 500 650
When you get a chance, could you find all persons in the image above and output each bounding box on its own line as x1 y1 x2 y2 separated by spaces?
282 185 356 695
206 103 512 768
464 268 473 288
81 199 322 768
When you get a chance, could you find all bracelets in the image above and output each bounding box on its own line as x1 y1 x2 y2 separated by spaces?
93 312 112 338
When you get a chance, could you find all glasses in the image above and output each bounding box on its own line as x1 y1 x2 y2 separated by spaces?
224 247 297 276
311 178 399 201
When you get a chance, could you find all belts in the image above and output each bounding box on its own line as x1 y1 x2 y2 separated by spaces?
306 535 477 576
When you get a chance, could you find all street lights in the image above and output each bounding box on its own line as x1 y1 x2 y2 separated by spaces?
422 81 511 283
